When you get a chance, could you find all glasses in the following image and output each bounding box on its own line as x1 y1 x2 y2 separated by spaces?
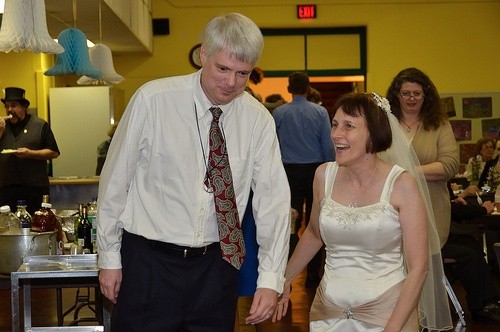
400 90 423 98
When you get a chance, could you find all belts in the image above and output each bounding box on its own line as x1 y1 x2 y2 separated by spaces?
130 234 215 259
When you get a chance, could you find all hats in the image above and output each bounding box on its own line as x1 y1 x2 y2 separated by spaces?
1 87 30 108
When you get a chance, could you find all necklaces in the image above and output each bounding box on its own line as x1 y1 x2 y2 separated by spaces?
345 157 379 208
193 101 226 193
400 118 420 133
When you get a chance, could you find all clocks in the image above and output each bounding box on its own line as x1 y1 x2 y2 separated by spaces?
188 44 202 69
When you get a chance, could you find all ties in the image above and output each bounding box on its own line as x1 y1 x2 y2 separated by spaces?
204 107 246 270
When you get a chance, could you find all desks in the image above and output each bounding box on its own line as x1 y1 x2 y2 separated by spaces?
448 180 500 273
49 177 99 215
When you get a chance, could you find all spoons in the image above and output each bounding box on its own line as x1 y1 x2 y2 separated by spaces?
475 191 482 204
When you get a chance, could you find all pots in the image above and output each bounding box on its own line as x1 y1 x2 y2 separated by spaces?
0 232 56 276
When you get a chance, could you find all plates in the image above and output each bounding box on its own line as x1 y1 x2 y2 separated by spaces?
1 150 17 153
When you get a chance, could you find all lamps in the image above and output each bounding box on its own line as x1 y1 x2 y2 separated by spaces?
43 0 103 79
77 0 126 88
0 0 65 55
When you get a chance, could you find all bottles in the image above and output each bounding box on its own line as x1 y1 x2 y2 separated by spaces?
74 203 83 244
0 206 22 235
15 201 32 234
77 206 93 253
88 195 97 240
43 195 48 204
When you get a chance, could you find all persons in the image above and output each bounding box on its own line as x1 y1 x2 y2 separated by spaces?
467 136 496 180
478 135 500 192
96 123 120 176
441 180 500 324
386 68 460 332
95 13 292 332
272 72 336 289
0 87 60 215
272 92 454 332
246 67 359 128
